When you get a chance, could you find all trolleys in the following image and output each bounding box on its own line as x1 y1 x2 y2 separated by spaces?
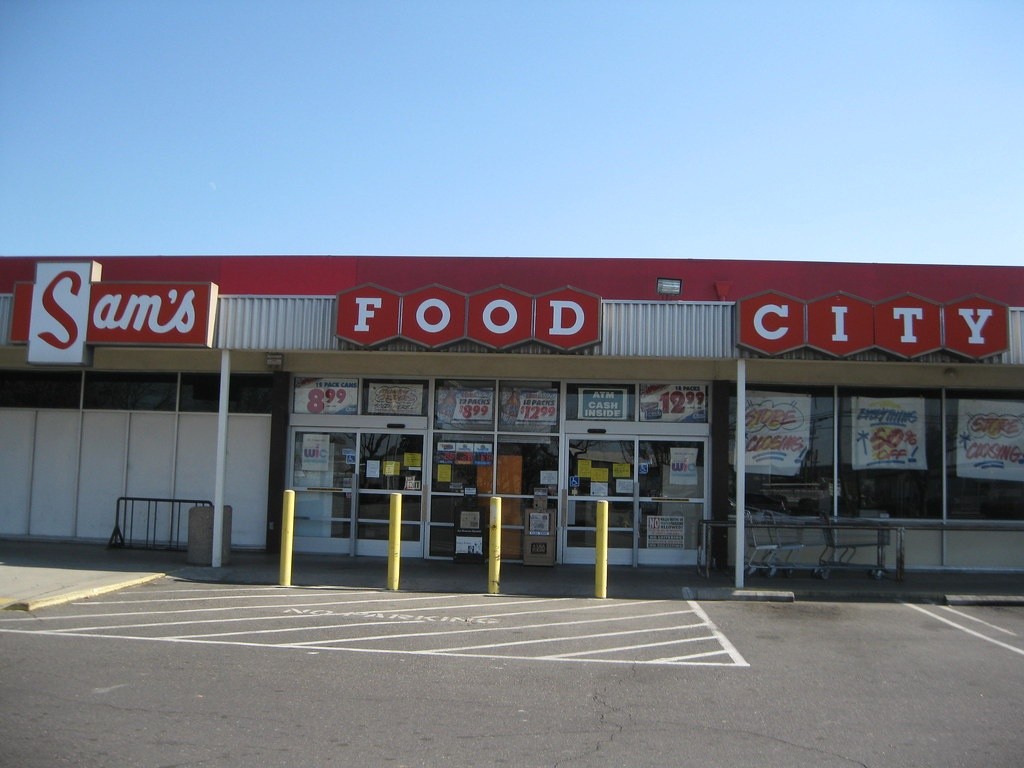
818 510 891 579
727 497 807 579
